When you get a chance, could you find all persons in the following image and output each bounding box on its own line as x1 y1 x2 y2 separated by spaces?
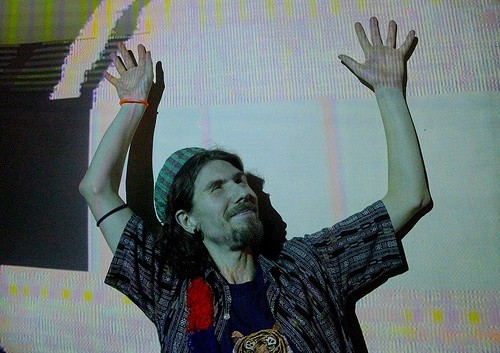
79 16 432 353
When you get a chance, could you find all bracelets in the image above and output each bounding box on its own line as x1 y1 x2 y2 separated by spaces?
119 97 149 109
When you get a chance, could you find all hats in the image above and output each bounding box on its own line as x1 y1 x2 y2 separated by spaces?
154 147 208 226
